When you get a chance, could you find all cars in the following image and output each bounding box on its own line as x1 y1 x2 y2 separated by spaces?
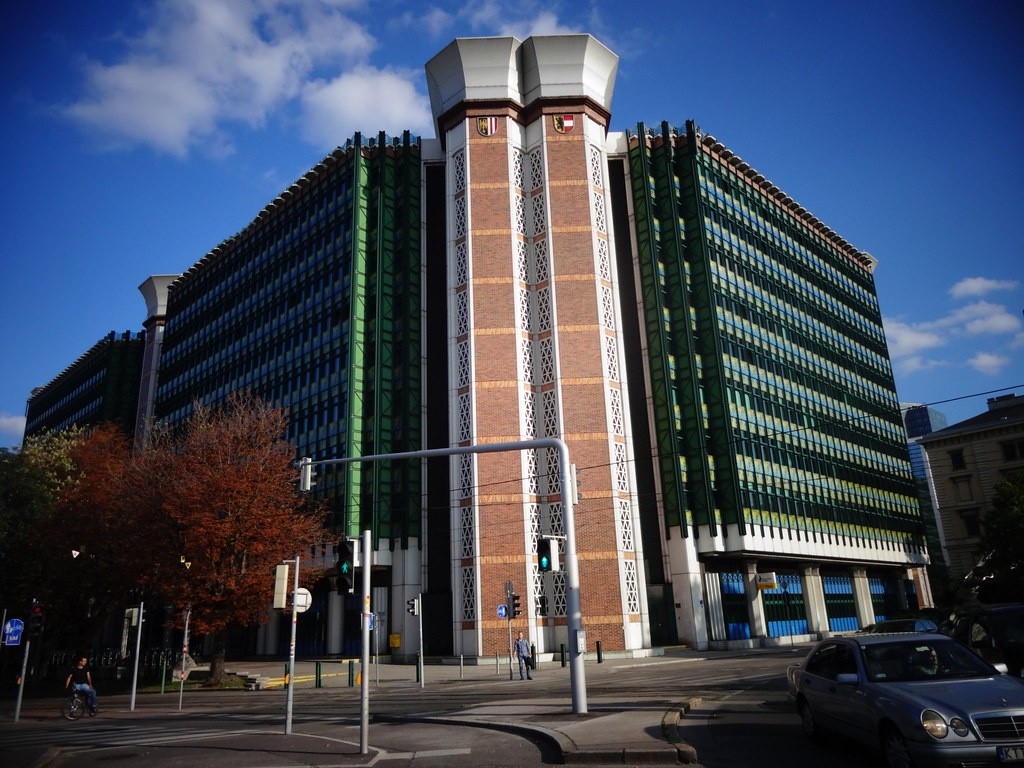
783 630 1024 768
871 619 940 633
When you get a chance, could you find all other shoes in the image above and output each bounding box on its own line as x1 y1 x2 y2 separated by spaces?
527 677 533 680
520 678 525 680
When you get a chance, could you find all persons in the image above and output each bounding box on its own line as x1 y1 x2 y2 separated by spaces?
512 631 533 681
61 656 96 714
913 608 939 635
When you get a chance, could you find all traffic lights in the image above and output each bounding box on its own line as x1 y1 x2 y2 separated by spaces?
337 540 354 595
302 460 317 491
537 538 560 572
270 564 278 604
509 593 522 619
407 598 419 616
27 603 44 642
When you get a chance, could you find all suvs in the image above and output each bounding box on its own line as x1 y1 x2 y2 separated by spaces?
936 602 1024 680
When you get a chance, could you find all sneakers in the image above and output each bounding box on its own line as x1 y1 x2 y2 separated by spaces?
89 704 97 713
68 713 76 718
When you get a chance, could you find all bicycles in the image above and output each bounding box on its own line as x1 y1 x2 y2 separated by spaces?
61 685 98 720
55 646 203 669
